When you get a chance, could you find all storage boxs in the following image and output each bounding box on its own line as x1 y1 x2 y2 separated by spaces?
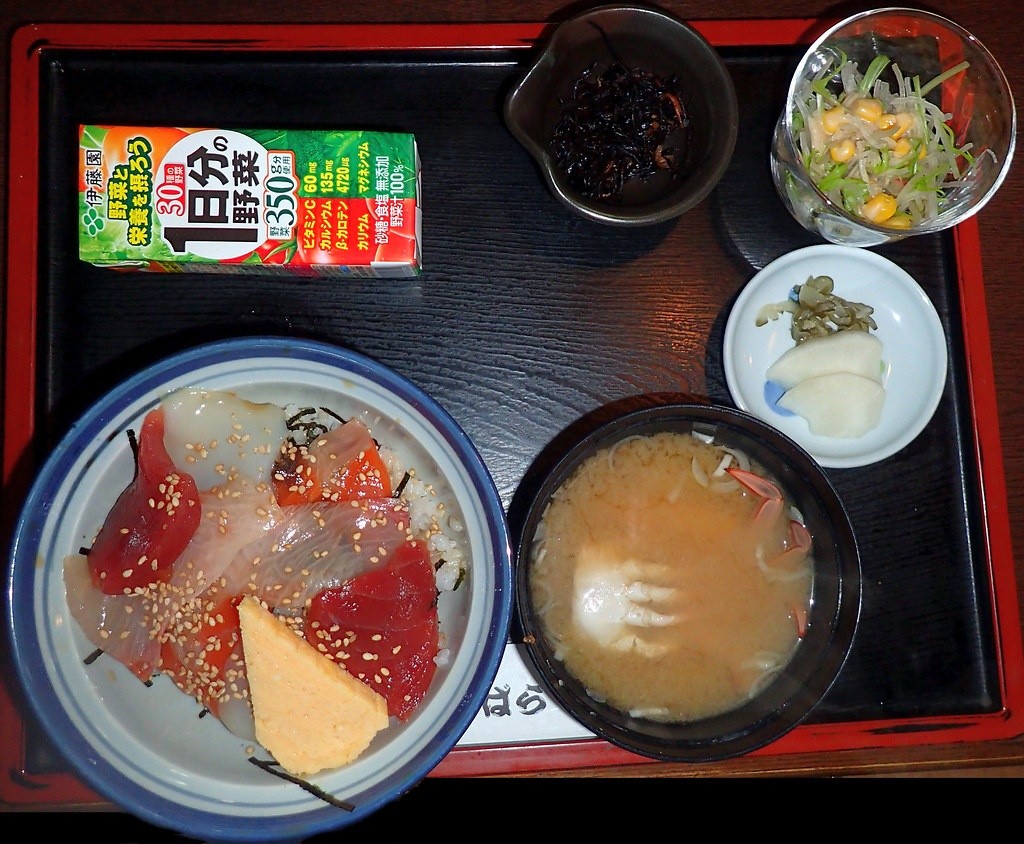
76 121 424 280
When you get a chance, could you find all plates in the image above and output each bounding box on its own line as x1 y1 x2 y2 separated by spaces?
722 245 951 470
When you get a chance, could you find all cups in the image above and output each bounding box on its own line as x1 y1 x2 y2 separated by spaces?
768 8 1016 247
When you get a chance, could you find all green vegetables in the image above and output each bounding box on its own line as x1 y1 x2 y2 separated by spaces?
781 50 976 226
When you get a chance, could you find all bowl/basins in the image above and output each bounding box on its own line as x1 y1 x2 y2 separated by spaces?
508 402 864 762
504 0 739 229
3 337 515 842
